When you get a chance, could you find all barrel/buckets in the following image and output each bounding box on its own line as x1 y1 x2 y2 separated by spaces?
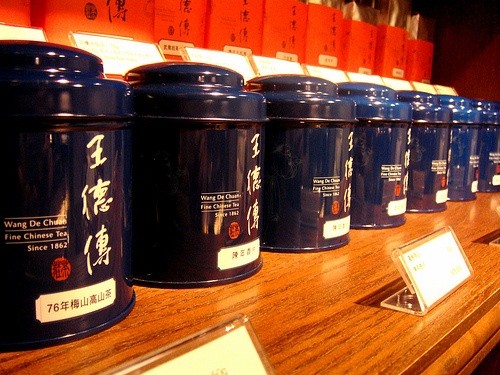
0 39 500 354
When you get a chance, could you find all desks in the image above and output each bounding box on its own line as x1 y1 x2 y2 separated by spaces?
0 190 500 374
